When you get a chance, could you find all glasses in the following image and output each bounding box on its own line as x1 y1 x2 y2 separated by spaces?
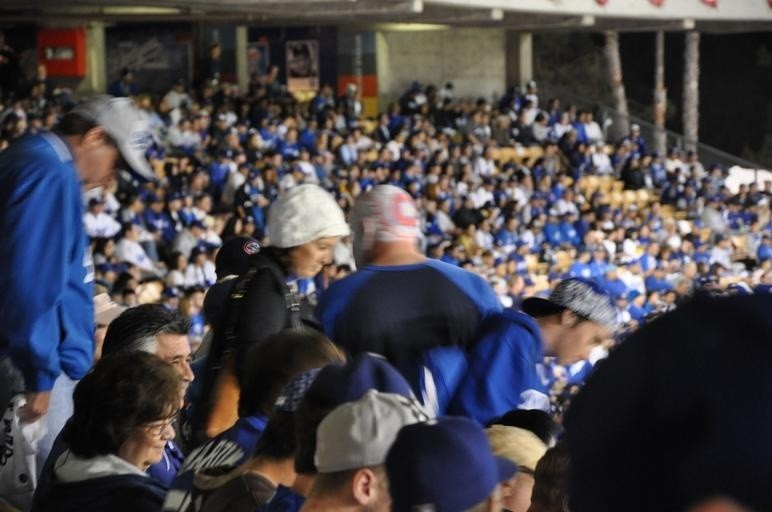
136 419 176 436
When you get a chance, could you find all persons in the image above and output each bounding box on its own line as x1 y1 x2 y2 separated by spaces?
0 45 771 512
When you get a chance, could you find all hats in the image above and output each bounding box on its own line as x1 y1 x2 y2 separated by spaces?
68 93 161 186
294 354 423 475
215 236 262 273
88 292 129 325
265 184 351 248
309 387 432 472
481 424 548 474
353 183 424 244
203 274 239 332
520 275 618 330
384 414 518 512
